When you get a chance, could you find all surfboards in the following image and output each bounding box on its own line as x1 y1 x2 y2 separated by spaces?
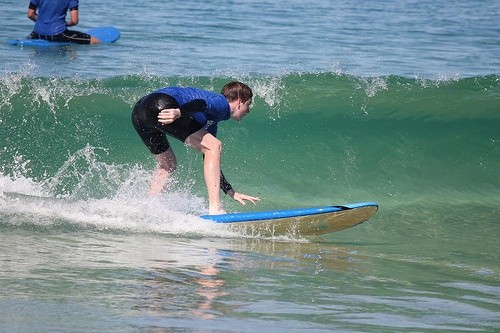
199 202 379 236
6 26 121 46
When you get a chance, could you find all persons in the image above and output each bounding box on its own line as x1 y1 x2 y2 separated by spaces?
131 80 262 215
25 0 102 46
